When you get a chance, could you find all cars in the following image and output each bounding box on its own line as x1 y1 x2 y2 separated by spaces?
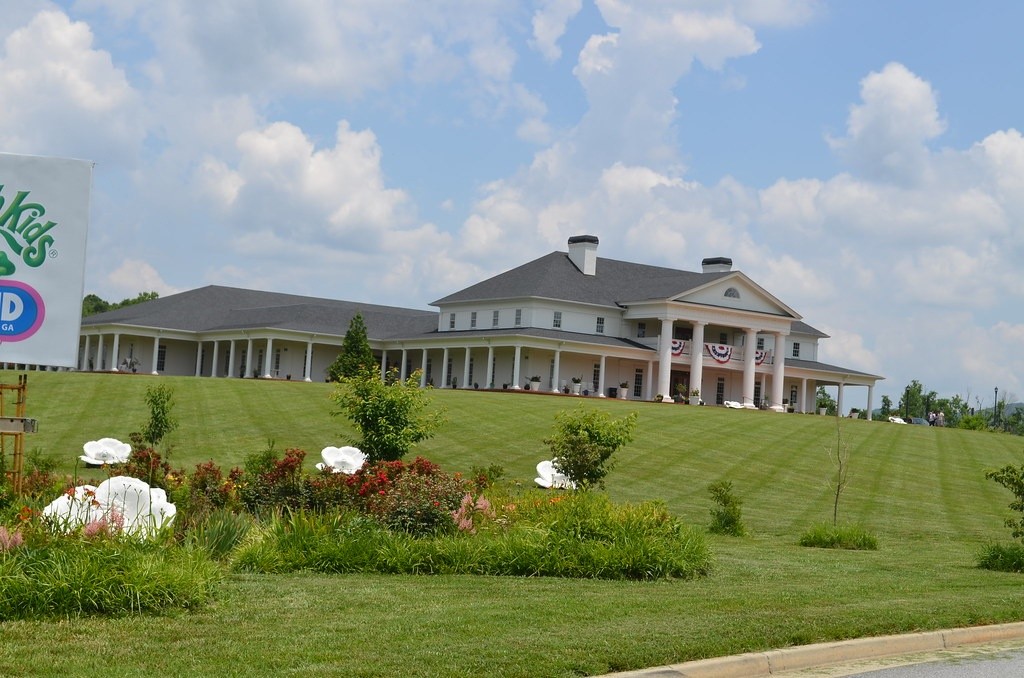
889 416 907 424
904 418 930 426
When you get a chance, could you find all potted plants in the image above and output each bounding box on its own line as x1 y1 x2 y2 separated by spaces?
819 403 827 415
620 381 629 399
690 388 699 406
851 408 860 419
783 398 789 412
452 377 457 388
674 384 686 405
572 377 582 395
530 377 541 392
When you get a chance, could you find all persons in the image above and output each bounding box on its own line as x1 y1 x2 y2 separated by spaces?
929 409 944 426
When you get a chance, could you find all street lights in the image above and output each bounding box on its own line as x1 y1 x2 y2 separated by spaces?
993 387 998 425
906 384 910 421
